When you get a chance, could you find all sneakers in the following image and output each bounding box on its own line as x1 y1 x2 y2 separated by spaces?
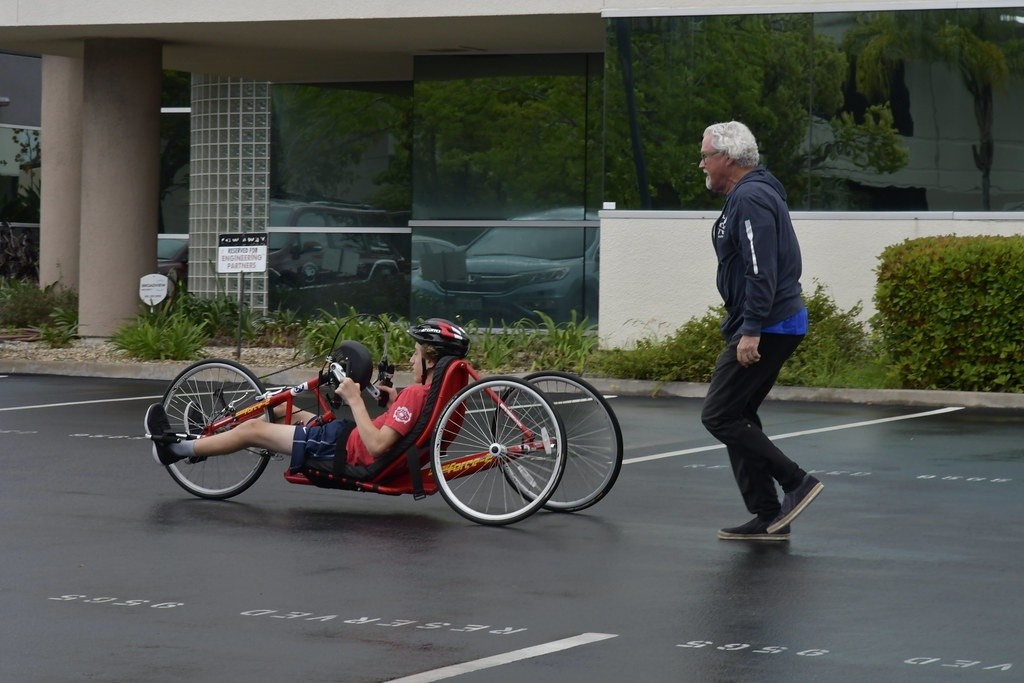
144 403 185 465
184 401 208 464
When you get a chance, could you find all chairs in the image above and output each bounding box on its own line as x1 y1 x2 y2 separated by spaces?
328 355 467 483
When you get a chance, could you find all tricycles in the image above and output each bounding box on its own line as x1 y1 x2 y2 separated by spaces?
145 315 626 528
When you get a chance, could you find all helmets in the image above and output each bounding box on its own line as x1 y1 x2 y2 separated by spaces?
406 318 470 358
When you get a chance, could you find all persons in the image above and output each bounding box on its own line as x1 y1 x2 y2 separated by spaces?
143 317 470 483
699 120 825 540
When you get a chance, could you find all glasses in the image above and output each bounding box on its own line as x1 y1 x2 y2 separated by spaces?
701 149 724 163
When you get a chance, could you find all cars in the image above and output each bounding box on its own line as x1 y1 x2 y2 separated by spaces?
159 201 468 330
439 203 600 324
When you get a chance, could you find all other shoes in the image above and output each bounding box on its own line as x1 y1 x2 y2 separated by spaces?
766 474 824 534
717 517 791 540
